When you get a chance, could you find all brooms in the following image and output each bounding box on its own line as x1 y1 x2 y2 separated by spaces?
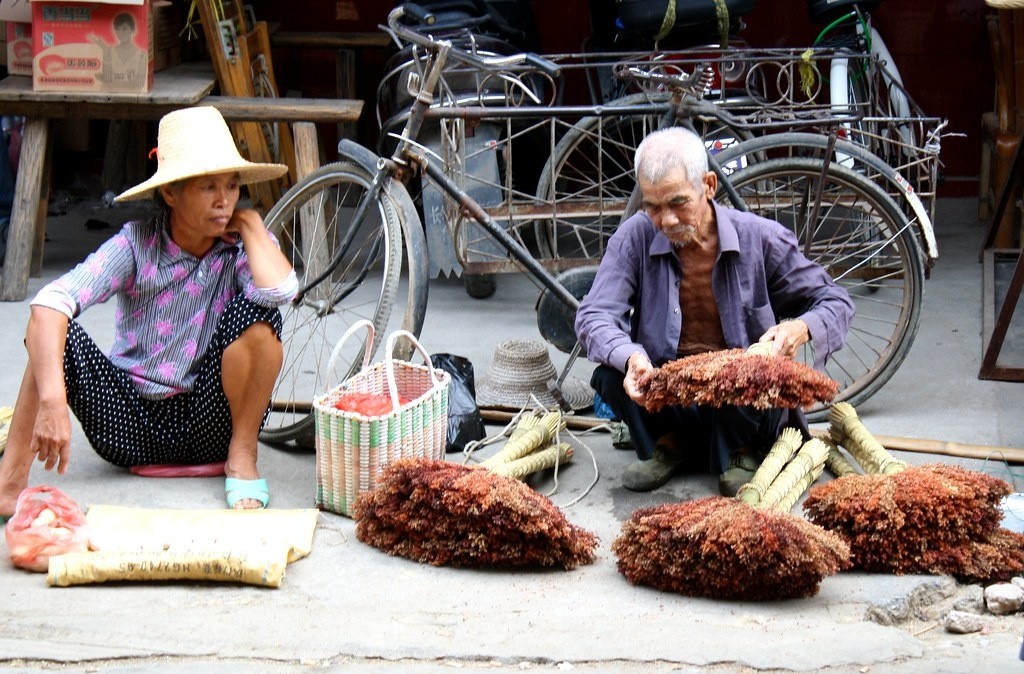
610 402 1023 603
351 409 600 574
633 339 842 411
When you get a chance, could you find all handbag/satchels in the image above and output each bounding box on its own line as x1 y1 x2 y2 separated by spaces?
6 485 87 572
420 353 487 454
336 391 409 418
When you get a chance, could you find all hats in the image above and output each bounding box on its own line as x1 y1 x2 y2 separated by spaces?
475 338 596 413
114 108 288 202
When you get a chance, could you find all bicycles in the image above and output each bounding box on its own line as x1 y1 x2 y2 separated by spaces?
241 0 946 447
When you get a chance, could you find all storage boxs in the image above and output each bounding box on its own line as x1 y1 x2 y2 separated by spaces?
0 0 183 94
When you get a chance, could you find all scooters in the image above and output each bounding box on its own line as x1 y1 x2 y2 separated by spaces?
376 0 558 299
581 0 762 214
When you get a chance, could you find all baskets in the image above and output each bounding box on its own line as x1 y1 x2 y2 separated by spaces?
313 320 451 519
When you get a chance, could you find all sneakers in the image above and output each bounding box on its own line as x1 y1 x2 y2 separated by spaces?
621 444 686 492
718 444 765 497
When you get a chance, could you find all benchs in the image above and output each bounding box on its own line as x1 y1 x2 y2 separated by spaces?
271 31 391 160
1 94 365 303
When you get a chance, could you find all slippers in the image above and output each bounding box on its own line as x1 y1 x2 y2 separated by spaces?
225 477 269 509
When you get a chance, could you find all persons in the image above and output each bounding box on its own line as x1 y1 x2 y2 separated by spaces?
95 12 146 94
575 125 856 495
0 106 299 525
